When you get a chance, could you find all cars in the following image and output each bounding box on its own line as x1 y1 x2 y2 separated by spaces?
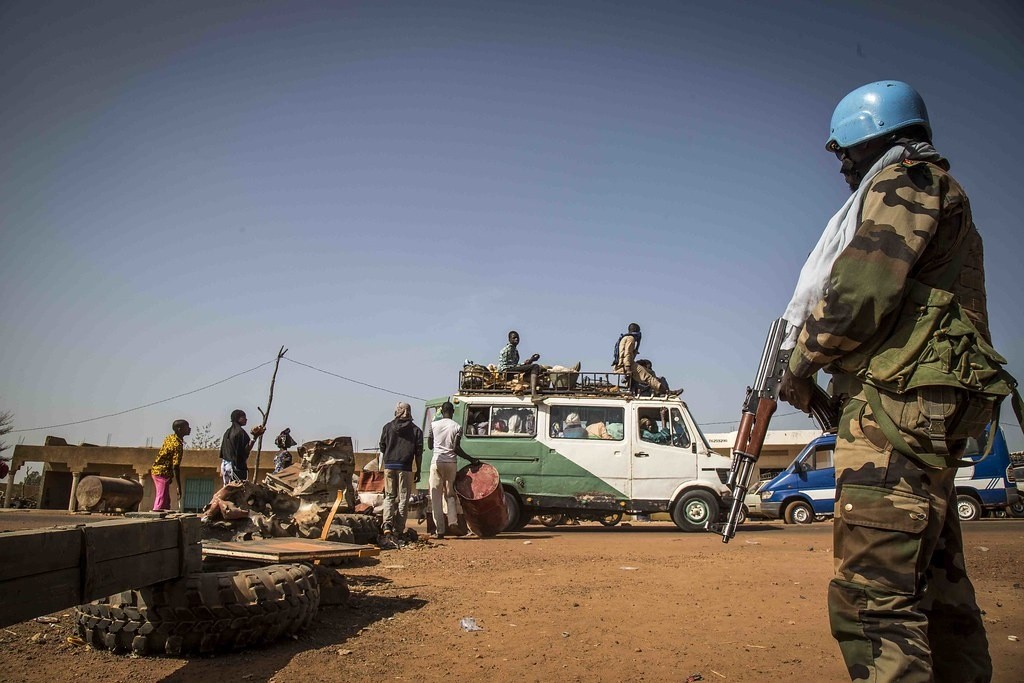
993 466 1024 517
728 479 827 522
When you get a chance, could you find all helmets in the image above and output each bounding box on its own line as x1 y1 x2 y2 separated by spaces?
825 80 932 151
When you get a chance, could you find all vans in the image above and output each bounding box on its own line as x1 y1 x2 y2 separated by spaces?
417 370 736 531
758 420 1018 527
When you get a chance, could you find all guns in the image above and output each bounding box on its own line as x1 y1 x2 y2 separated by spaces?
720 317 842 545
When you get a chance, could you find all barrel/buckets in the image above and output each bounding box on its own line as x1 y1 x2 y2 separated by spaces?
76 476 143 512
453 463 510 538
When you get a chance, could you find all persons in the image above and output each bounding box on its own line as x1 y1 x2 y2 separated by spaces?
219 409 260 484
428 403 480 538
611 323 684 396
778 81 1019 683
379 401 423 537
467 408 534 434
499 330 548 402
640 407 671 443
151 419 191 509
274 428 298 472
550 408 623 439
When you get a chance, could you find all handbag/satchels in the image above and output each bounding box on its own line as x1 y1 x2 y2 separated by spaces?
836 271 955 388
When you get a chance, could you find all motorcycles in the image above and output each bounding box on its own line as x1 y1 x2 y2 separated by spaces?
538 514 623 527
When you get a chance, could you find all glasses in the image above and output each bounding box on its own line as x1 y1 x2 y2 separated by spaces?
833 143 849 162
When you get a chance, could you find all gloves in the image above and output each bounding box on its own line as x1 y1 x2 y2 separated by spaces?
779 366 815 415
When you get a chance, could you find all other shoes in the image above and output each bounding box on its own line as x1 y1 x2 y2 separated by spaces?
531 395 549 403
450 523 464 536
438 532 445 539
382 523 392 535
667 388 683 396
394 529 403 538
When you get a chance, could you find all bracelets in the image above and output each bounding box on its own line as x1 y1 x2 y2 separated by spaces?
251 439 255 441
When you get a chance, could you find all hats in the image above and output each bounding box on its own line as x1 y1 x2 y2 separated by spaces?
639 418 649 427
565 413 581 426
395 401 412 418
473 410 483 420
